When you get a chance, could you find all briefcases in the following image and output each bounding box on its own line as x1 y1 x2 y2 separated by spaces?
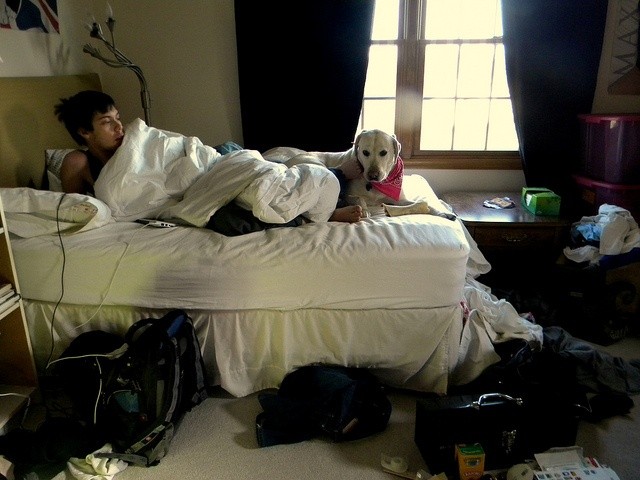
416 388 579 475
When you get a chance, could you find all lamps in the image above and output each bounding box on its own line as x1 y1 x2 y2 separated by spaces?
78 2 151 127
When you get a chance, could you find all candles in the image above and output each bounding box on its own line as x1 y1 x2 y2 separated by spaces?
104 2 115 26
81 22 96 36
88 13 99 27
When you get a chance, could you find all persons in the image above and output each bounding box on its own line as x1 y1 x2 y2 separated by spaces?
54 90 365 234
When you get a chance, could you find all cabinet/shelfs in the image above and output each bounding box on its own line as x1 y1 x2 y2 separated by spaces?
0 193 39 398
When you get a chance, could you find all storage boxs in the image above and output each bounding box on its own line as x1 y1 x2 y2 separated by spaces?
575 175 639 226
579 111 640 186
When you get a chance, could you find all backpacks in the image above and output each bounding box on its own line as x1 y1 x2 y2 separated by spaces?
93 310 210 466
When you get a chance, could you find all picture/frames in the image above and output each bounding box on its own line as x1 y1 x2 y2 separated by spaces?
591 0 639 115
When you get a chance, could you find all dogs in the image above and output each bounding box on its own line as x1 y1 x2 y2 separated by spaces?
284 129 402 218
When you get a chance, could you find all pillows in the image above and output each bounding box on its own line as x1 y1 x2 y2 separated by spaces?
1 186 110 237
44 145 90 192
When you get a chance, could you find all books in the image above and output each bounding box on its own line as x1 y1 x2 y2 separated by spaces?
0 282 12 296
0 289 15 306
0 294 21 315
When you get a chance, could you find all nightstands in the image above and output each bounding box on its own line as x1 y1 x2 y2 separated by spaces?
444 190 573 317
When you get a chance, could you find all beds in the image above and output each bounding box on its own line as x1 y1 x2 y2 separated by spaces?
0 70 479 397
